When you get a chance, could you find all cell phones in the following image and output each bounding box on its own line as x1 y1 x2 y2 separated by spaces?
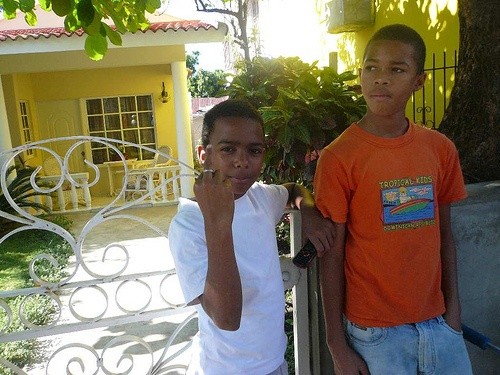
293 240 317 268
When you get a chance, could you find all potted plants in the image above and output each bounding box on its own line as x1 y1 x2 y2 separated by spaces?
104 145 130 160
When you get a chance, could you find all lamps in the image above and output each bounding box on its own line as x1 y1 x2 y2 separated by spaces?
159 81 171 104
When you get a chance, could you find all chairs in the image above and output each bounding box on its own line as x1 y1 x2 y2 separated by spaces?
43 154 91 209
122 145 176 199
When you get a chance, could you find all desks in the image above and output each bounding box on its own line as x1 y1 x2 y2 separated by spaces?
102 158 138 197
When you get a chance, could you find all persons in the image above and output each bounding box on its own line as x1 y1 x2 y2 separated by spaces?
313 24 473 374
168 99 335 375
